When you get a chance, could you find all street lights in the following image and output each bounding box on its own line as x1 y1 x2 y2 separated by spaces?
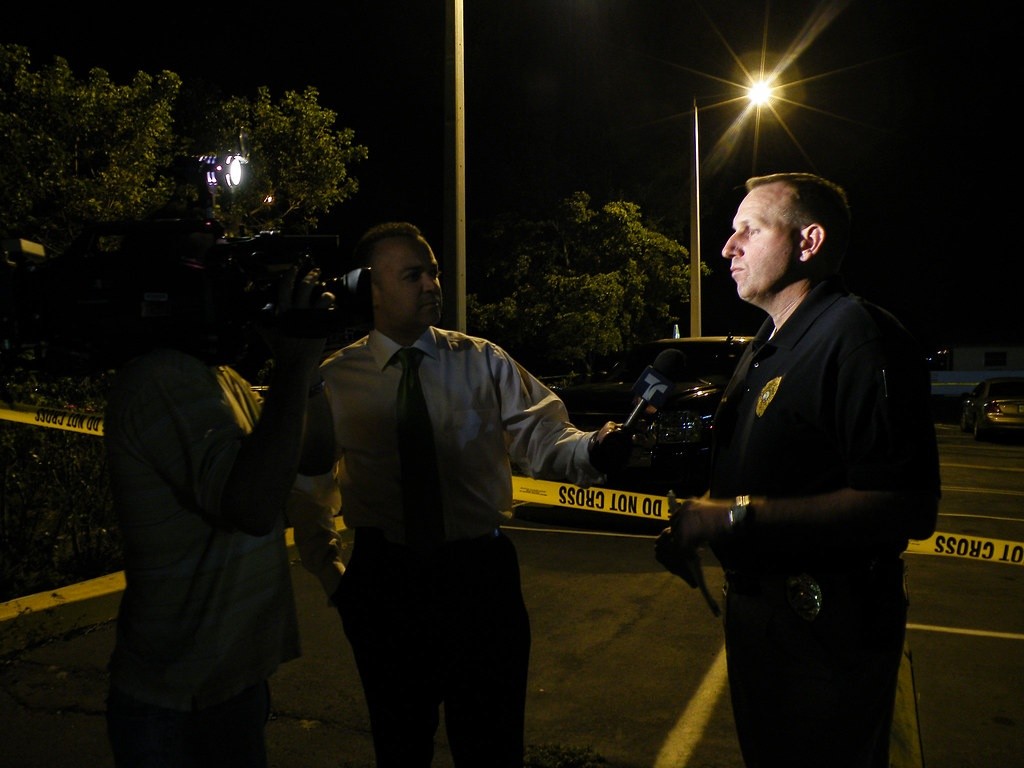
689 60 791 338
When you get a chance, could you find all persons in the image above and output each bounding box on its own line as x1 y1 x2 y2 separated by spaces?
654 171 942 768
104 208 330 768
290 221 629 768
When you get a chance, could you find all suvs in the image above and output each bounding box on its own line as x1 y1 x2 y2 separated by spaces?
632 333 757 494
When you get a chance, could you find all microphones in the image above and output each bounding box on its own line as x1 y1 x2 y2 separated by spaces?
217 230 340 255
621 348 687 433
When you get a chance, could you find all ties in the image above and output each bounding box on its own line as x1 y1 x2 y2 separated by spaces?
389 346 448 557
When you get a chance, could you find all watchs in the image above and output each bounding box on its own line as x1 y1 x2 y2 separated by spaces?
727 495 754 536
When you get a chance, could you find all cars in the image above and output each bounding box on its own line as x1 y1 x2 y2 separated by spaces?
961 376 1024 442
